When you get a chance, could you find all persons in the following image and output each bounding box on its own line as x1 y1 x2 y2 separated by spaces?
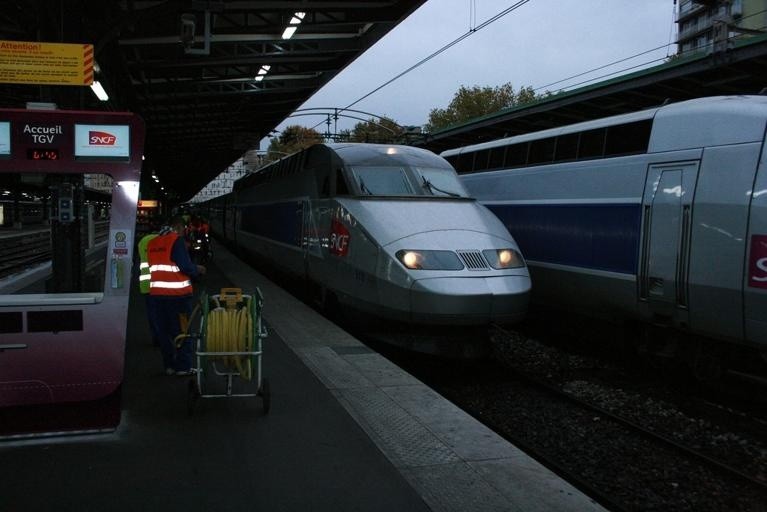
138 205 211 379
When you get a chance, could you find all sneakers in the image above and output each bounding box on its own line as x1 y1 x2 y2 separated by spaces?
165 367 203 377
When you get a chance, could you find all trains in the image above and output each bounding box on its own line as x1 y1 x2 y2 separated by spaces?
434 93 767 343
190 136 533 343
